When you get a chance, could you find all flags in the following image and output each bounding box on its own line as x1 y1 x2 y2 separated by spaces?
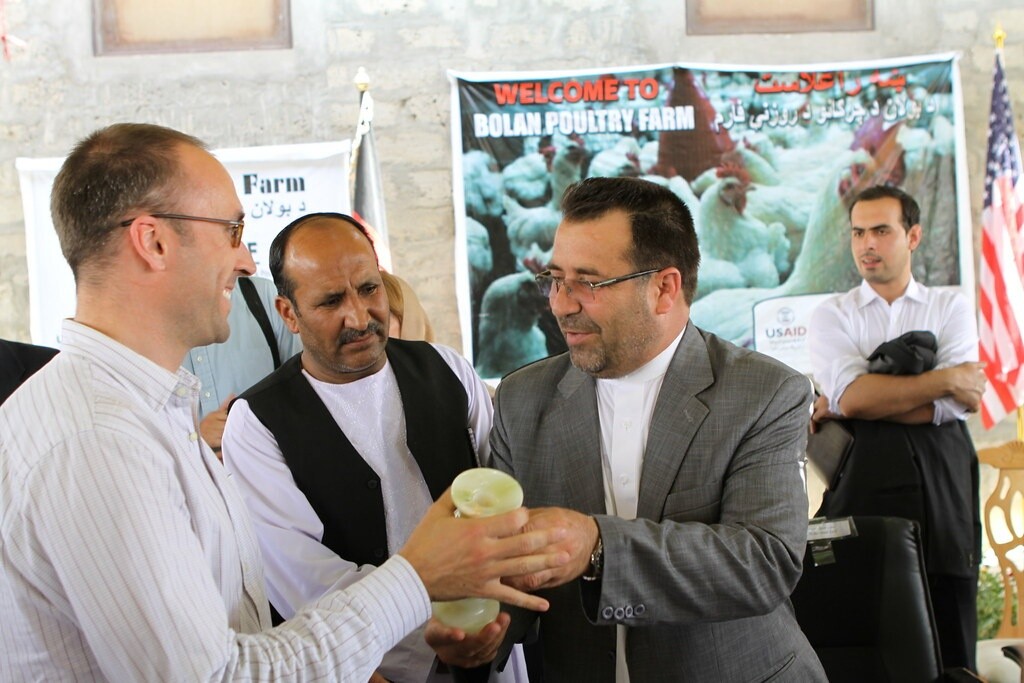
978 50 1024 432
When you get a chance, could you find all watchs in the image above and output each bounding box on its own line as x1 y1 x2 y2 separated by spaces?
582 537 603 581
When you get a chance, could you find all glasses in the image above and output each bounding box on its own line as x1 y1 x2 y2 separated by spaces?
534 268 661 301
121 207 244 248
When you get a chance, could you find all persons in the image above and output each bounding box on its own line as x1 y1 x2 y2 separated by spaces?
380 269 435 343
425 178 829 683
809 185 987 683
0 123 570 683
222 213 529 683
182 277 303 466
0 339 61 407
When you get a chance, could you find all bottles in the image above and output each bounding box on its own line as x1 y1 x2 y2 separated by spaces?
431 468 524 636
